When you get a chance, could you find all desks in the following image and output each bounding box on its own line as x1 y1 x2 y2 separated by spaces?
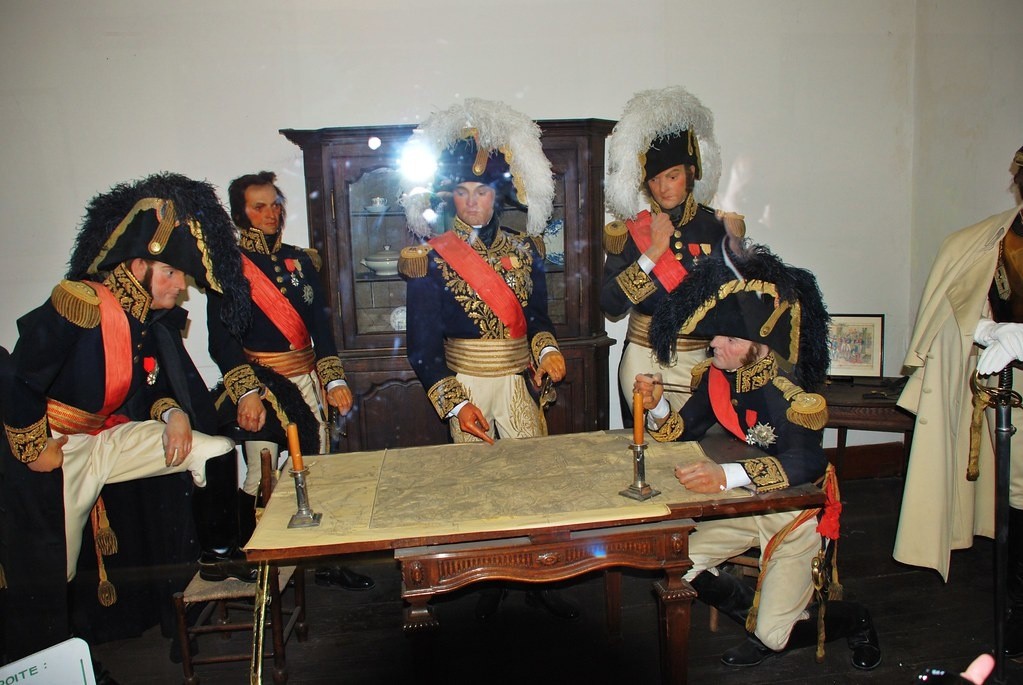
817 376 917 483
241 425 829 685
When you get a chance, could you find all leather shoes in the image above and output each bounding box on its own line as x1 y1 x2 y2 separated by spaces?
720 637 790 668
481 584 509 619
198 545 261 582
524 586 580 622
314 564 375 591
849 618 882 671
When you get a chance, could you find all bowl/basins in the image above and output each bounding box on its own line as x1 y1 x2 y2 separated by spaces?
364 246 400 276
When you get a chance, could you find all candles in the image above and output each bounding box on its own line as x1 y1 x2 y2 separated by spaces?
286 420 303 471
632 389 644 444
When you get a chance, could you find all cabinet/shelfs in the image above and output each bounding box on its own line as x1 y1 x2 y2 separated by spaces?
277 117 619 453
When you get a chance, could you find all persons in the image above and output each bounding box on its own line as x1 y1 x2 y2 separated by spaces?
397 98 580 622
205 171 374 645
633 252 884 670
592 90 747 433
0 174 267 685
893 144 1023 658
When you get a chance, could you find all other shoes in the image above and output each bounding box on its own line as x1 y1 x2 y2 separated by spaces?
170 632 197 664
1001 605 1023 657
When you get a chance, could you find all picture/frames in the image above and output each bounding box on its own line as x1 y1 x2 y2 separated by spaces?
825 313 885 381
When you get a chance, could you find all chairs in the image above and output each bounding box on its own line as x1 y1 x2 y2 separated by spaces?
172 449 312 685
709 463 845 662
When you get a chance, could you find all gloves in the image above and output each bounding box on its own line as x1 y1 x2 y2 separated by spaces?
974 318 1023 375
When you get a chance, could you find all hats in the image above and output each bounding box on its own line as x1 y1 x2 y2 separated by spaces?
647 246 832 384
64 173 251 304
606 88 722 221
399 98 555 236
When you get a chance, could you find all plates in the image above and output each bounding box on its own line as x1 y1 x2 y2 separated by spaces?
362 207 390 212
542 220 565 267
390 306 407 330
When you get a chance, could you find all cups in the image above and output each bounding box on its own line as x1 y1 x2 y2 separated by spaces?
371 196 388 207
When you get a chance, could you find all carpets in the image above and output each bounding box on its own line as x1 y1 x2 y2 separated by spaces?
822 442 904 480
